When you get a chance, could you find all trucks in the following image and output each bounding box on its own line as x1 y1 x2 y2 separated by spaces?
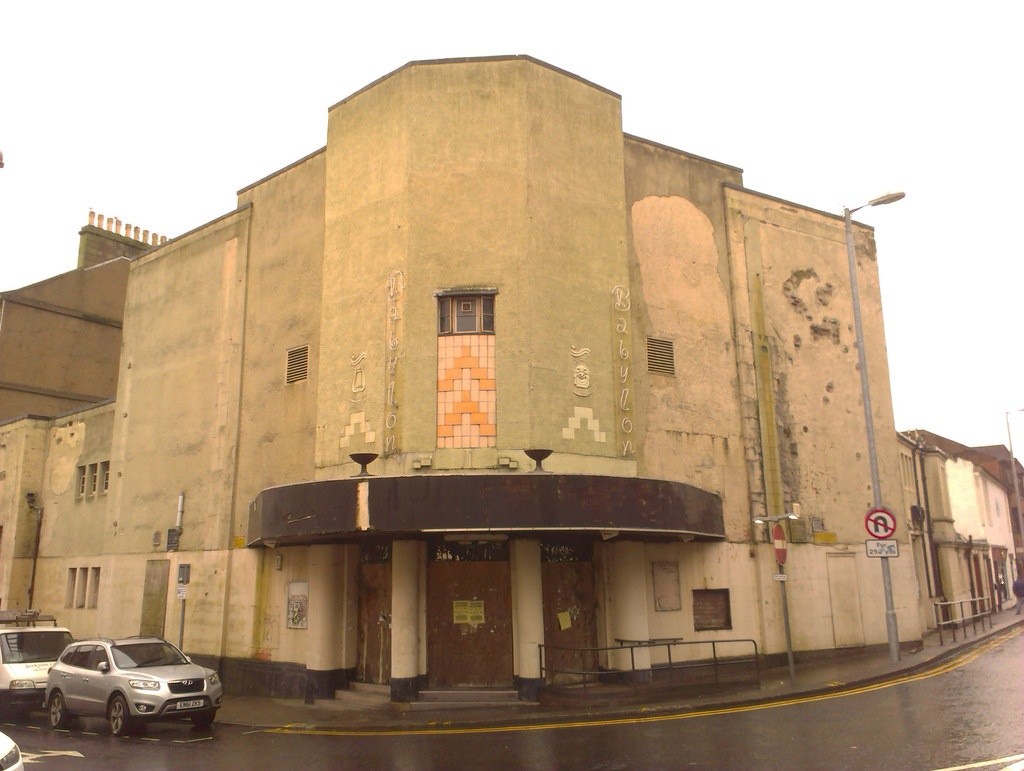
0 609 76 726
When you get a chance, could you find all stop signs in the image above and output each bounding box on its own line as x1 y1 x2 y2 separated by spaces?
772 522 788 566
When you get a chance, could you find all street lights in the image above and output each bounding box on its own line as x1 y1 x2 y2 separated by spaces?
751 512 801 678
845 191 909 663
1005 408 1024 556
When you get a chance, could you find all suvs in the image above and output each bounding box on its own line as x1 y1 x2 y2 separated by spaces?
45 634 222 738
